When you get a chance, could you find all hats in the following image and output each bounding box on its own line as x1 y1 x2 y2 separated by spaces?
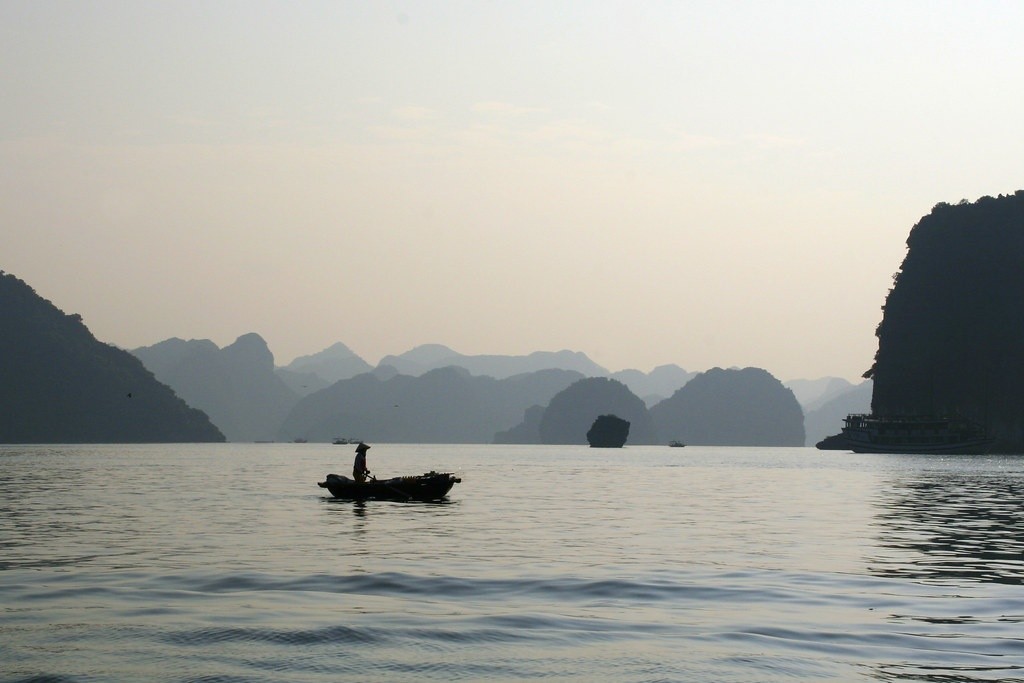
355 443 371 452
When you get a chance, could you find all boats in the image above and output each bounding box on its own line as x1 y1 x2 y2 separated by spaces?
316 469 462 502
839 411 1003 455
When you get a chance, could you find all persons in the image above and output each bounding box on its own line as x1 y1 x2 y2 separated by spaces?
353 443 370 484
